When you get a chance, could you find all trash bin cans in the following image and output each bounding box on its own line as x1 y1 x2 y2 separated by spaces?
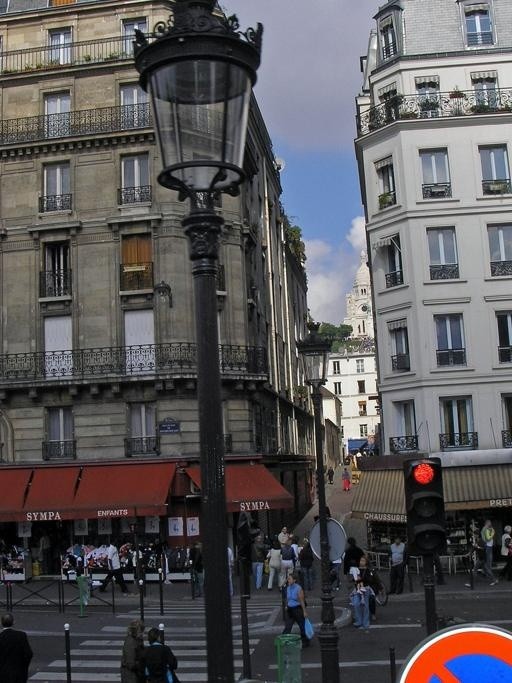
76 576 91 618
273 634 303 683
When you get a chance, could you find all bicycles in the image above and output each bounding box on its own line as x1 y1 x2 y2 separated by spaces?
344 562 389 606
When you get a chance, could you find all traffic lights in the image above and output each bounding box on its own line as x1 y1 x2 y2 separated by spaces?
403 455 448 559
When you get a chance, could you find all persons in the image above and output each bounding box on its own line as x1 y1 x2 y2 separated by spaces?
227 546 235 596
138 627 180 683
189 540 203 598
251 526 315 593
386 535 407 595
38 533 50 574
99 536 131 595
282 574 311 649
0 612 33 683
120 619 144 682
465 520 512 589
341 468 351 491
326 466 335 484
343 536 380 636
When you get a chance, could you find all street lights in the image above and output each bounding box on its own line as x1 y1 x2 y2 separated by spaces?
126 1 265 681
288 320 347 680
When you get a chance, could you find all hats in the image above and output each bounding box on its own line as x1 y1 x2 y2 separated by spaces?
128 621 145 636
148 629 160 643
